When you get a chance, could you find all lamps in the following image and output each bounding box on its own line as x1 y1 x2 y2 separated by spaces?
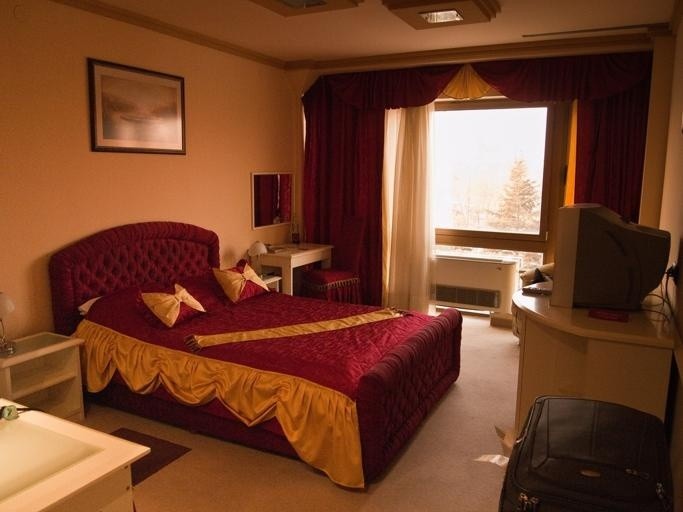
0 291 14 352
383 1 501 31
256 0 360 20
247 241 268 280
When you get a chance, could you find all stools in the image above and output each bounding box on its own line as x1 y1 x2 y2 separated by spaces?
302 268 361 307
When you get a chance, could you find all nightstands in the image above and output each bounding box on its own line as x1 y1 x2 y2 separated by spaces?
256 273 282 292
0 331 86 423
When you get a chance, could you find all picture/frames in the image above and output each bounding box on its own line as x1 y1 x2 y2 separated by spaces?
85 57 186 156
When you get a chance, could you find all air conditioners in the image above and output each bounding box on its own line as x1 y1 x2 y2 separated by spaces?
427 254 519 316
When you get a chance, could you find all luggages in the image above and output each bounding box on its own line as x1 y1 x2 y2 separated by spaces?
496 393 675 512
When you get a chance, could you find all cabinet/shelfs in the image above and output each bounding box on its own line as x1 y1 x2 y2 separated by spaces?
502 283 675 452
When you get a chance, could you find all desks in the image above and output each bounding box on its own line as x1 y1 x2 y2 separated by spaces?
0 396 151 512
253 241 335 298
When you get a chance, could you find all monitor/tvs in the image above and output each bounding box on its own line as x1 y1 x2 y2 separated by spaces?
550 203 671 308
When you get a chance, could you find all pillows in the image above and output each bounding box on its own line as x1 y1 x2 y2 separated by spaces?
209 257 271 308
131 281 206 330
76 295 102 316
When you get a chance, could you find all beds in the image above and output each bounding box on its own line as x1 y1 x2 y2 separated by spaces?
44 218 463 493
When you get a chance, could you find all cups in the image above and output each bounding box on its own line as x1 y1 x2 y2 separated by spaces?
291 223 299 244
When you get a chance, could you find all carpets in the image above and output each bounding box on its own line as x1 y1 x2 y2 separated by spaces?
110 427 191 488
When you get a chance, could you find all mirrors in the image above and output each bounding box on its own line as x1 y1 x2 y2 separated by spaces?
251 171 296 230
